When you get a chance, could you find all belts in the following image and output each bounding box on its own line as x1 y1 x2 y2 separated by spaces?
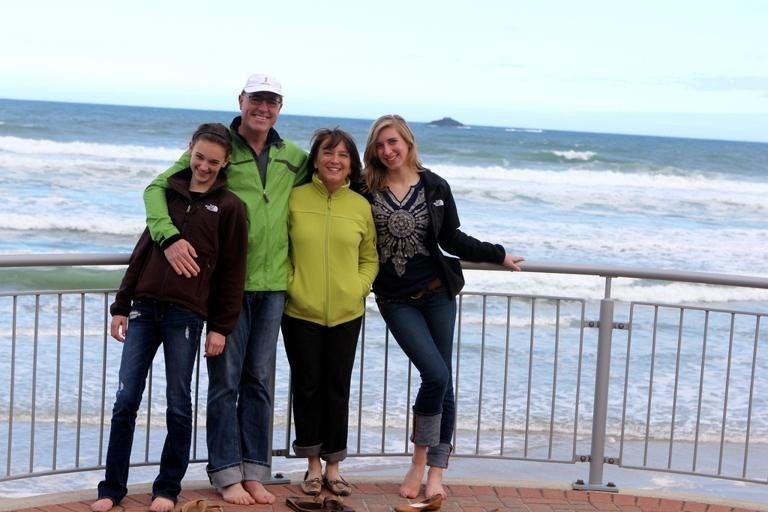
410 279 441 299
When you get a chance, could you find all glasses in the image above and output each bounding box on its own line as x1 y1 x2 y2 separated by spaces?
243 94 282 107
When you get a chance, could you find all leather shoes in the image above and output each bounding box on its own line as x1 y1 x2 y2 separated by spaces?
395 494 442 512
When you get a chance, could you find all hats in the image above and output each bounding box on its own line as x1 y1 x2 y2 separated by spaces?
241 75 285 98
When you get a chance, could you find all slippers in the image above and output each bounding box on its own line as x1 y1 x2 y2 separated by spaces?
286 496 324 511
181 499 223 512
325 495 354 512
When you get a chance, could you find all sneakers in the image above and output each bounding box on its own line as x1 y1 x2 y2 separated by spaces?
301 469 322 496
323 472 352 496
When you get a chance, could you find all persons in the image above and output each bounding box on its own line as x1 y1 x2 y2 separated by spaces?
281 126 381 496
348 114 524 502
90 122 249 512
143 72 317 506
